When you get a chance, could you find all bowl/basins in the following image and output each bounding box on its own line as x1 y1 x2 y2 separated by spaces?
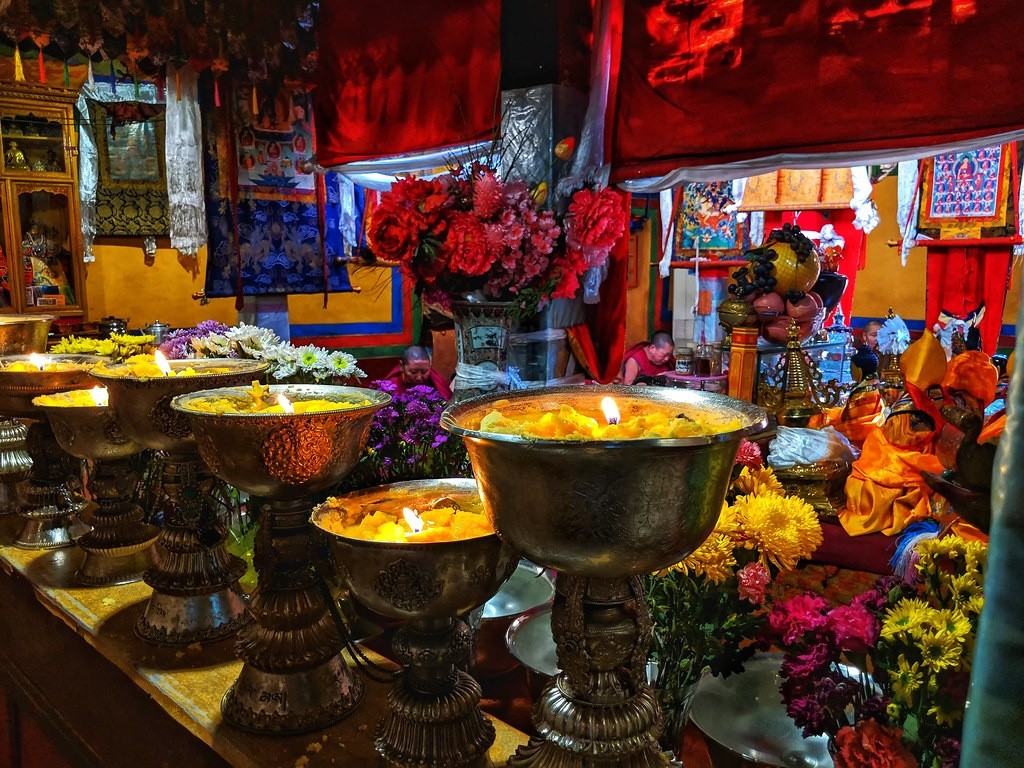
477 560 556 651
504 603 553 704
688 652 883 768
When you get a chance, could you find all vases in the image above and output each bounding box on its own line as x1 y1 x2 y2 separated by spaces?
443 302 519 407
647 660 703 762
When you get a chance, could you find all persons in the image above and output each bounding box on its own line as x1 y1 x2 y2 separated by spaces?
6 141 25 163
618 333 676 385
842 345 885 420
382 345 452 400
863 321 883 348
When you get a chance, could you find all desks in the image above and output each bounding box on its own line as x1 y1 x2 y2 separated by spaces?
0 504 713 768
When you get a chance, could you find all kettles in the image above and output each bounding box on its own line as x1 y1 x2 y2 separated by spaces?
139 320 170 344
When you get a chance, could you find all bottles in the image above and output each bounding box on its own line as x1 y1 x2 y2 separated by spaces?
694 342 722 377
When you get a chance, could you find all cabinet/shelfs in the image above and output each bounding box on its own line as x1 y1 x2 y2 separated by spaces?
0 78 93 331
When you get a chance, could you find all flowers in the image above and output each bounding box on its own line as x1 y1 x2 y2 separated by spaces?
639 438 824 755
363 134 630 320
